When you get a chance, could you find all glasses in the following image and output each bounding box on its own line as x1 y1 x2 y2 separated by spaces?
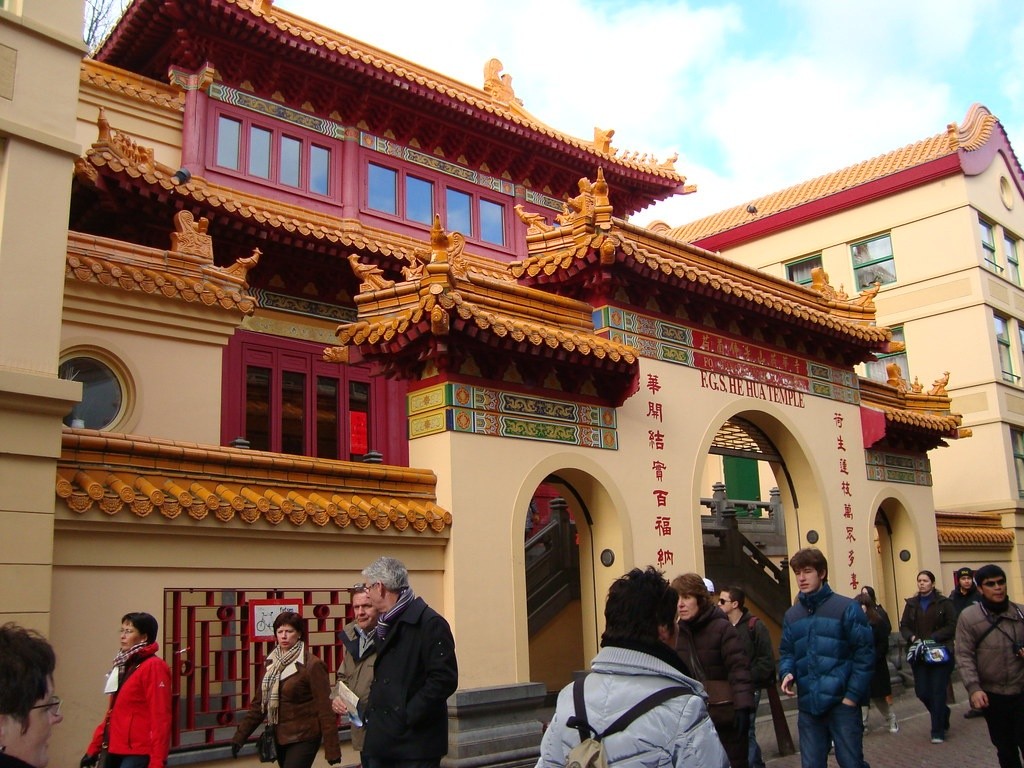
718 598 731 604
118 629 135 636
24 695 62 717
363 583 376 596
981 578 1005 587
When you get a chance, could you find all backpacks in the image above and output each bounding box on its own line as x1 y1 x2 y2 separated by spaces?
566 675 694 768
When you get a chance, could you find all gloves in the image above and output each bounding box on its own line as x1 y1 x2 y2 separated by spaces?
327 757 341 765
79 753 100 768
231 744 241 757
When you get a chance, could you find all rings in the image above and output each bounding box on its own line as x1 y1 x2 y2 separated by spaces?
335 708 338 710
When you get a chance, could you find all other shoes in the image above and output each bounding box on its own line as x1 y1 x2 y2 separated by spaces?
931 736 943 743
965 710 983 719
889 719 899 733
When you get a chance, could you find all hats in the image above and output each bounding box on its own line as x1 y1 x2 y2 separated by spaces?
704 578 715 592
957 567 974 580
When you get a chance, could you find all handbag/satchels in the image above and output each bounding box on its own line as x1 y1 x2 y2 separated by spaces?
255 729 278 764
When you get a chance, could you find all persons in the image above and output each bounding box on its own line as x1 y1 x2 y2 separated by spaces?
332 585 382 763
534 565 731 768
363 558 459 768
778 548 876 768
232 612 342 768
955 564 1024 768
900 570 958 742
855 586 898 733
672 573 775 768
0 621 63 768
80 612 172 768
949 567 983 718
